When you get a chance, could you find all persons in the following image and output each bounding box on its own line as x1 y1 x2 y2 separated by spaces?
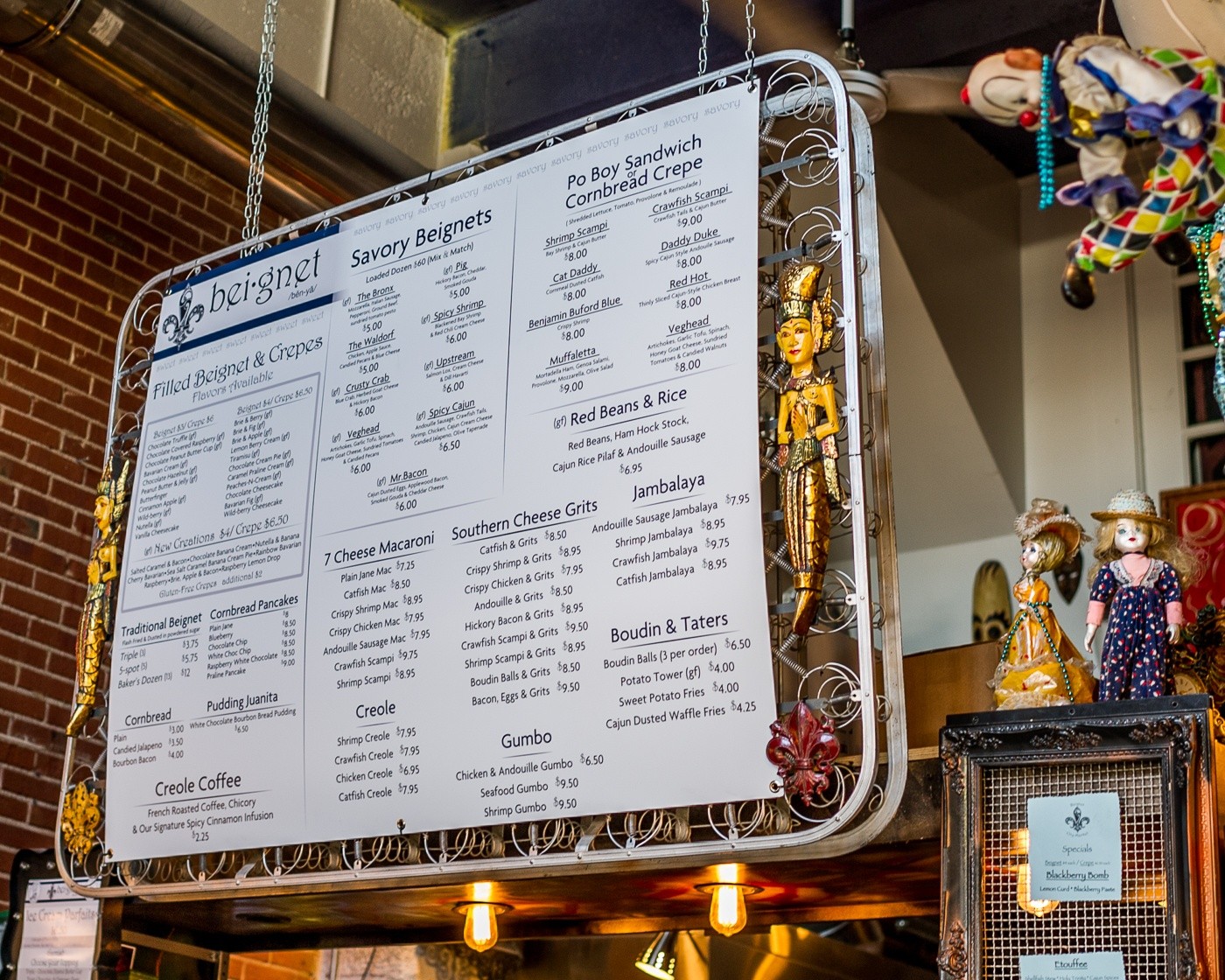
985 497 1099 712
776 259 847 636
960 33 1225 423
1084 488 1184 702
971 559 1012 644
65 455 130 739
